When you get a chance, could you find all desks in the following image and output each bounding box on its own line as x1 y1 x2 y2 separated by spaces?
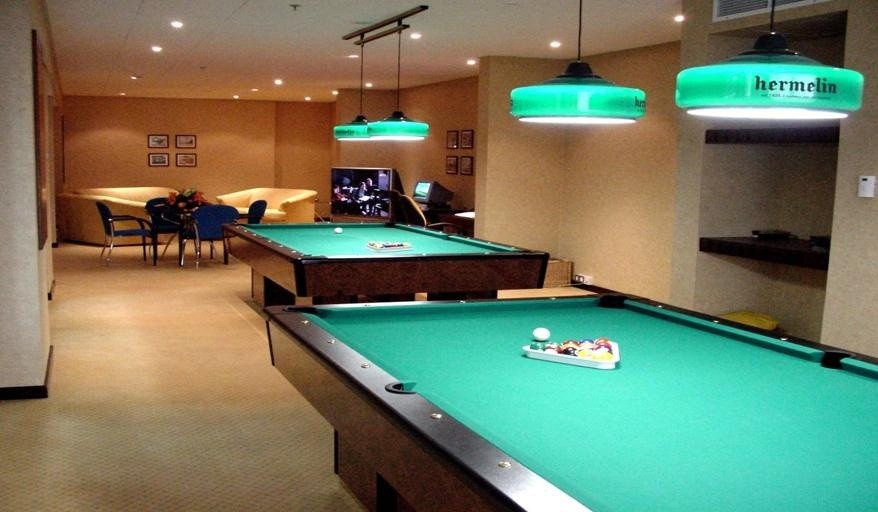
455 212 475 218
161 214 191 266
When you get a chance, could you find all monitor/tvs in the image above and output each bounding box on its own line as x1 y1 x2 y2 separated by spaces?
332 167 393 220
412 179 453 204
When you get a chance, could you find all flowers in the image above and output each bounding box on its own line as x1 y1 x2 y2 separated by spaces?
167 188 208 211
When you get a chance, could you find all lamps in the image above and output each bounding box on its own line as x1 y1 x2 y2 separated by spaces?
676 0 865 119
367 19 429 142
333 33 368 142
510 0 647 123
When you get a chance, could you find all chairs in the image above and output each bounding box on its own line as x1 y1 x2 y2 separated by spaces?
210 200 267 260
145 198 201 258
391 190 461 235
181 205 239 265
96 202 157 266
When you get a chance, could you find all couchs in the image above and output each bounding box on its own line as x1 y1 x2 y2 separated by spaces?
58 187 180 246
216 188 317 224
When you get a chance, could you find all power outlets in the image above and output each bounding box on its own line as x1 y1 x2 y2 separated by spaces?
857 175 875 198
576 274 592 286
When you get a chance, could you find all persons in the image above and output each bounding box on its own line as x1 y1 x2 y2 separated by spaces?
367 178 373 186
352 182 368 203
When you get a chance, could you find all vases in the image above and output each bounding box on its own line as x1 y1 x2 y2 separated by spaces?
181 212 192 220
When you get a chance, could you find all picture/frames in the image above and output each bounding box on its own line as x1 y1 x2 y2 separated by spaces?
461 130 473 148
176 153 197 167
175 135 196 149
32 28 49 251
460 157 473 175
447 131 458 148
148 153 169 167
446 156 458 174
148 134 168 148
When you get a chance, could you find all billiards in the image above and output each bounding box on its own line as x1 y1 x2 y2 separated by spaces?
562 344 579 351
530 342 545 352
594 343 611 349
580 339 594 345
578 350 592 358
592 346 612 354
595 337 608 344
544 346 558 355
532 327 550 341
592 351 613 361
563 347 576 356
563 339 578 347
544 343 562 354
578 346 594 350
368 240 404 249
335 227 342 234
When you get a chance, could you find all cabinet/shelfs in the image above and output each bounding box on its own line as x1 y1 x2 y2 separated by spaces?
330 215 387 223
